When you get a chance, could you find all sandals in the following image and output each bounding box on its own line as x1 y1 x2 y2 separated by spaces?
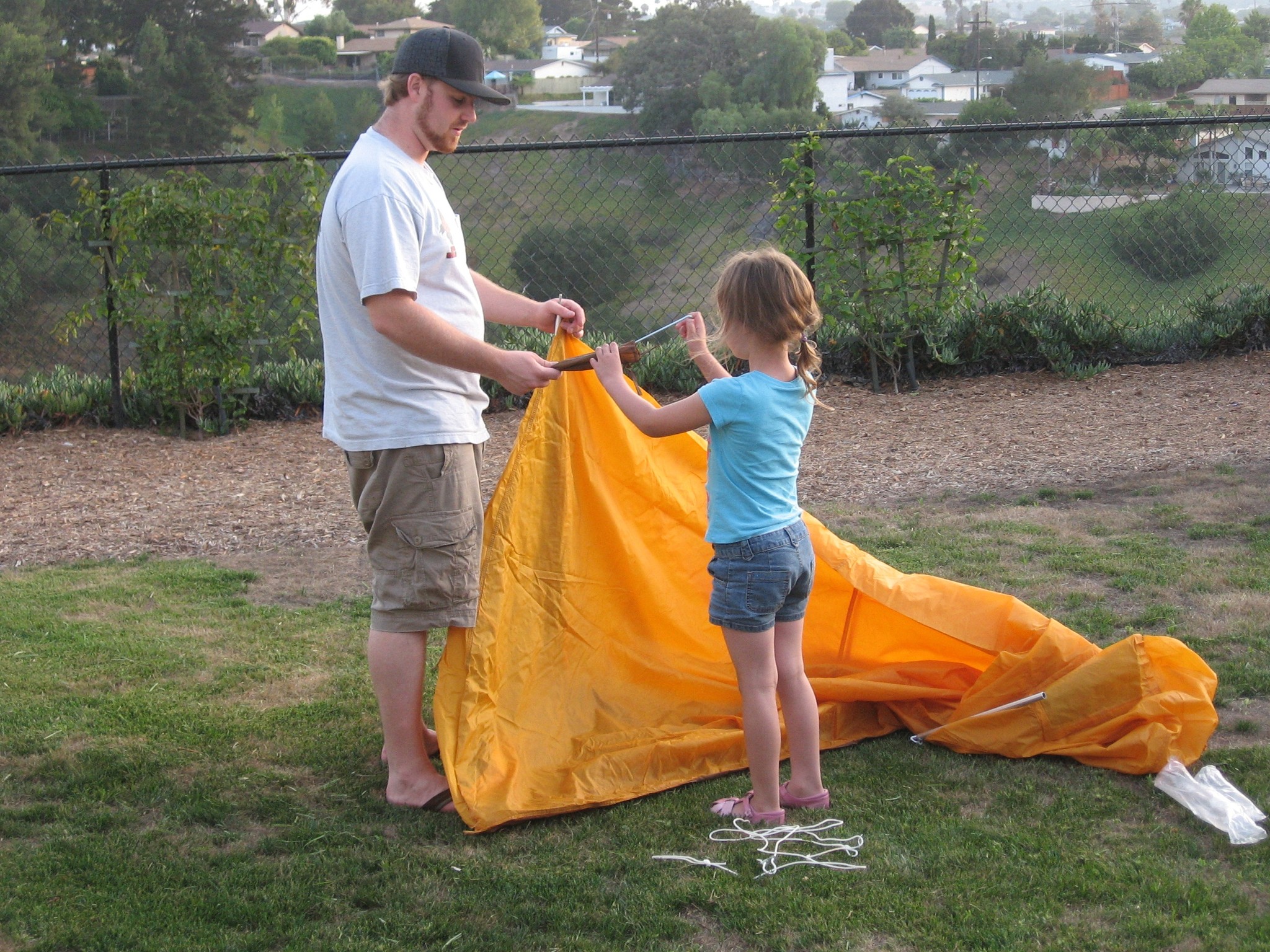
779 779 830 813
709 789 785 828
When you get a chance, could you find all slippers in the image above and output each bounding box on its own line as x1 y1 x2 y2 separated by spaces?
425 787 457 813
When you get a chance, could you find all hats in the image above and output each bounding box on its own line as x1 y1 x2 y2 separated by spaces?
392 27 511 106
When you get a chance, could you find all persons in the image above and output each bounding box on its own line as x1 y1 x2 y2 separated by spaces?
311 25 586 817
588 245 831 824
